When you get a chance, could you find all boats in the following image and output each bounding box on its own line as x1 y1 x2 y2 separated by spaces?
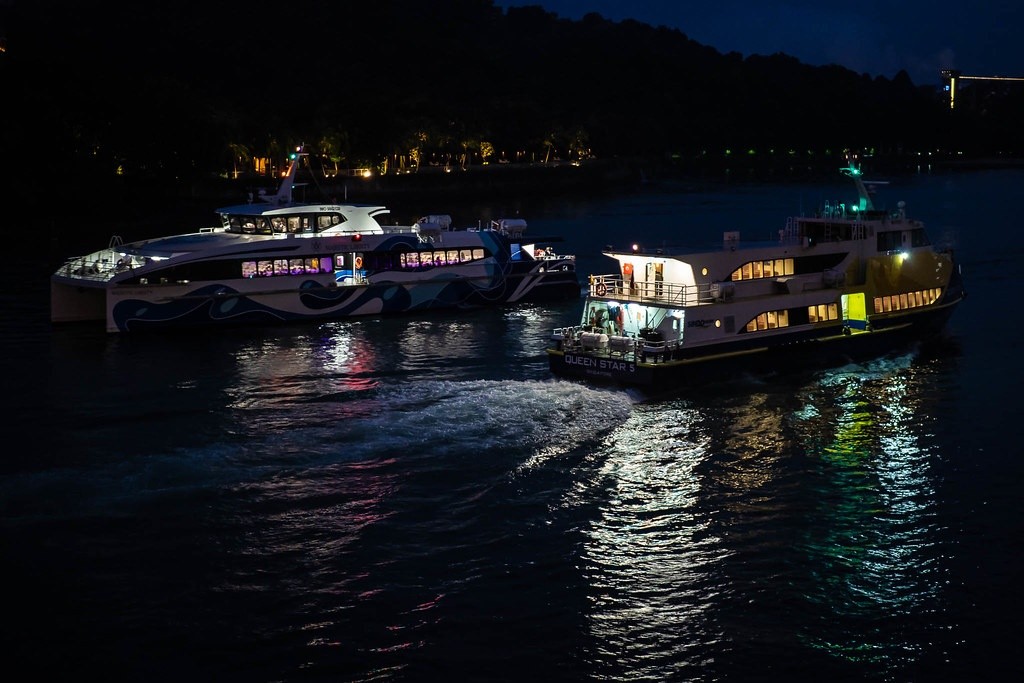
547 158 967 385
49 144 577 334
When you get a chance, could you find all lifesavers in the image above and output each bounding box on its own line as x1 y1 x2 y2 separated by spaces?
596 283 607 296
355 256 363 269
